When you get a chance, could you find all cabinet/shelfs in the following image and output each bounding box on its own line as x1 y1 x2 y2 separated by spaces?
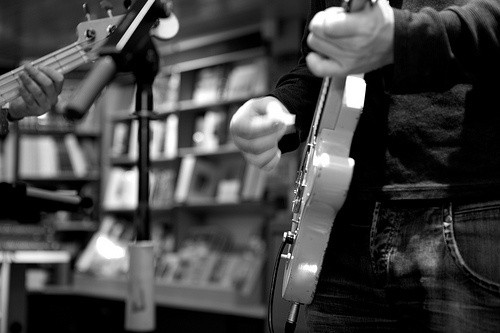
100 27 282 321
0 70 101 251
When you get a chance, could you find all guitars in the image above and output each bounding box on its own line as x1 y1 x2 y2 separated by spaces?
280 0 377 307
0 0 179 111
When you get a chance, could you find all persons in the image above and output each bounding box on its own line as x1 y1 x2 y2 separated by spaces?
231 0 500 333
0 64 64 143
